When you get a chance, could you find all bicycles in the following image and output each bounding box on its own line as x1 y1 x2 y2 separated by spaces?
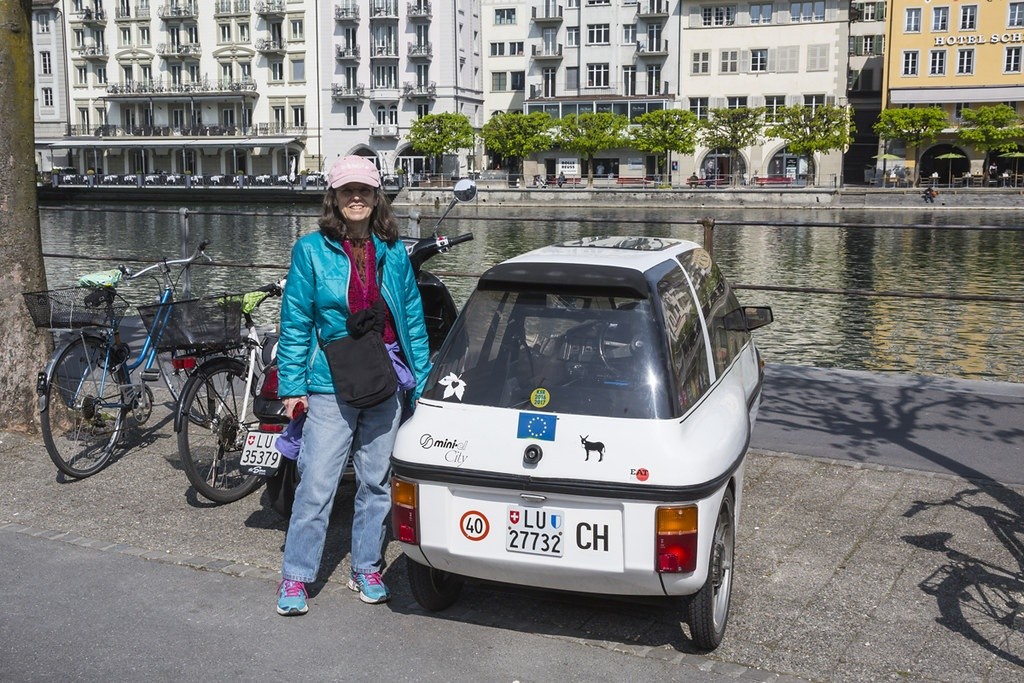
21 240 287 503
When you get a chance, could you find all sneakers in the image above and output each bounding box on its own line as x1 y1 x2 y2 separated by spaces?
347 570 391 603
275 579 308 615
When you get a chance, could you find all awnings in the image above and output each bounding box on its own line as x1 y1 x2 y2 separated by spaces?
46 137 300 188
890 86 1024 104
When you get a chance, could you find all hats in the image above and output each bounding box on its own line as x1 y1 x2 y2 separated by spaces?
329 155 382 189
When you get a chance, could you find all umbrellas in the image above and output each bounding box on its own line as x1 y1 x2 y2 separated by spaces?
871 153 904 160
934 152 966 188
997 151 1024 187
288 157 296 190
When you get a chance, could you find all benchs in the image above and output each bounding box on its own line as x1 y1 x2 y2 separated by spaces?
687 178 725 187
547 178 581 189
754 176 792 187
617 177 651 189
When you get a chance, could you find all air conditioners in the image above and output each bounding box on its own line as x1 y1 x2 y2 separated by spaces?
369 123 398 136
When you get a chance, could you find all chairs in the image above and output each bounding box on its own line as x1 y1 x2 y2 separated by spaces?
971 175 983 187
952 174 964 188
886 174 901 187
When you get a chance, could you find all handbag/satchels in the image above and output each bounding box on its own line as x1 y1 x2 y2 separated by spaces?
324 324 397 407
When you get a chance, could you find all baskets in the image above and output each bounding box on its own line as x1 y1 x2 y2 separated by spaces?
135 292 244 353
23 285 130 328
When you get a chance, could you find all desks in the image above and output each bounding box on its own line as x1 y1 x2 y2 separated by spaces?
962 176 973 188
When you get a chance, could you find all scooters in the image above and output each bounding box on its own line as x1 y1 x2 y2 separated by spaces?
239 180 481 519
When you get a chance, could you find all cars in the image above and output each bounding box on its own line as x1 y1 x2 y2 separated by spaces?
386 236 775 650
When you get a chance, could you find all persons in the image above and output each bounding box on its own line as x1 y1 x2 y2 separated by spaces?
704 172 713 189
705 160 714 174
275 155 433 617
689 172 698 189
558 172 565 188
989 162 997 178
